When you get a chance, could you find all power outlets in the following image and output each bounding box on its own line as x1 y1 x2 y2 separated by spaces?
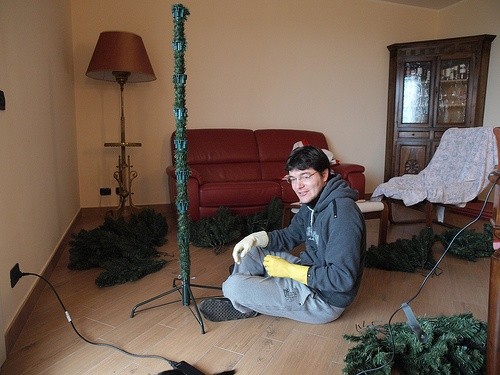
10 264 22 288
100 188 111 196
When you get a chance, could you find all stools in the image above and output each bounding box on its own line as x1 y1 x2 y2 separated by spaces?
281 200 388 248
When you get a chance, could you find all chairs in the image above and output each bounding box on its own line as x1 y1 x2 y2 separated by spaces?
371 127 500 247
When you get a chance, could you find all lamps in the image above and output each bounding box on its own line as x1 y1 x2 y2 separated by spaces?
85 31 157 225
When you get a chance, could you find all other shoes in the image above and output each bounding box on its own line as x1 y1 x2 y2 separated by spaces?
200 295 259 322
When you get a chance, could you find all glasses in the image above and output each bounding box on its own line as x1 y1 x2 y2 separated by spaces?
286 171 318 184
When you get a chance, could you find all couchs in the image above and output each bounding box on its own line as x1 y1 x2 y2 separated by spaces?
166 129 366 223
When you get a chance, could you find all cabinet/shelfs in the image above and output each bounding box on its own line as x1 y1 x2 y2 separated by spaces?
384 34 497 182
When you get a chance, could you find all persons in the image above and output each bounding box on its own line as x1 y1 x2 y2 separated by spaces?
198 145 368 325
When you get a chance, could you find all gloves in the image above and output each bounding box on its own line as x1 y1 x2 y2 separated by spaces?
263 255 309 285
232 231 269 264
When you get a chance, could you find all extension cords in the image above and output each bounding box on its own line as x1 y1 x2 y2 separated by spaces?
174 360 205 375
